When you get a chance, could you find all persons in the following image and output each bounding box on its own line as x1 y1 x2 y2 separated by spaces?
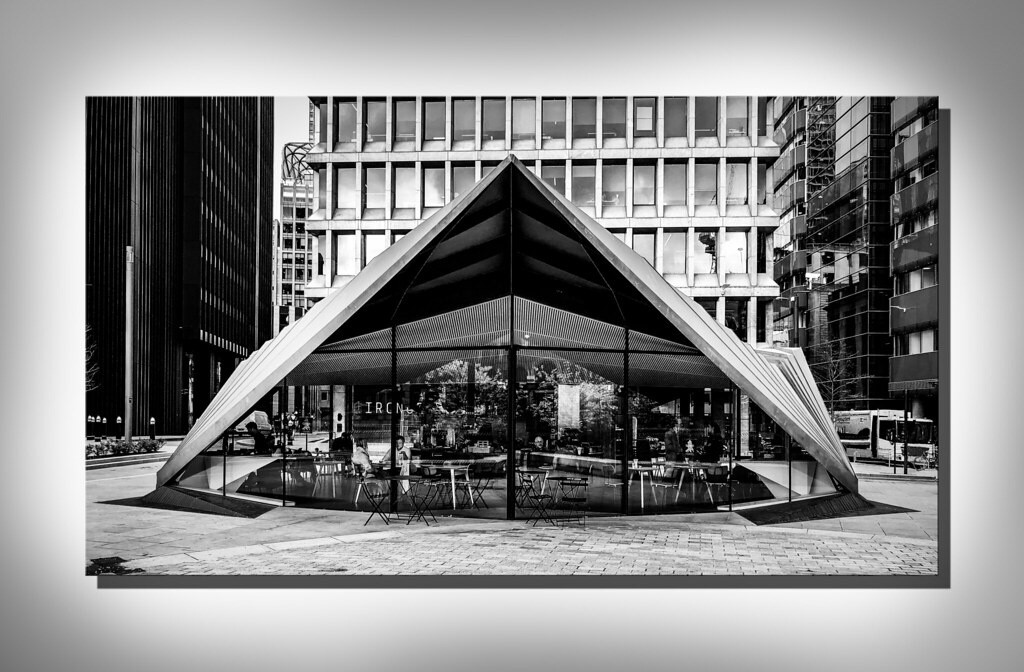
527 437 550 495
265 434 306 484
330 432 356 479
352 435 389 499
664 416 694 484
550 439 580 503
244 422 270 457
695 419 723 481
379 435 411 496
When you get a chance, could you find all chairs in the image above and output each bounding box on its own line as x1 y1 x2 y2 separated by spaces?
312 451 589 532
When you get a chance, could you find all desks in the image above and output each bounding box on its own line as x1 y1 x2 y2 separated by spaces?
628 466 658 507
675 465 716 504
354 464 474 510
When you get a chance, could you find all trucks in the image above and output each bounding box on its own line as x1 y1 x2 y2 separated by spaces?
235 410 272 436
829 409 936 467
